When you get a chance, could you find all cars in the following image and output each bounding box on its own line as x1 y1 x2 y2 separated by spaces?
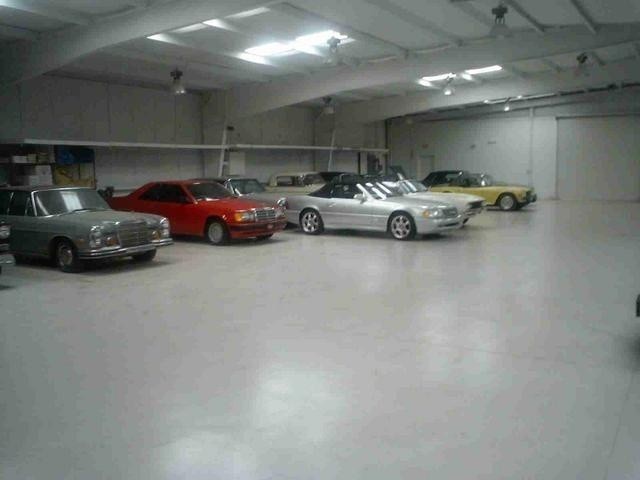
106 175 288 244
267 171 537 242
1 186 174 273
